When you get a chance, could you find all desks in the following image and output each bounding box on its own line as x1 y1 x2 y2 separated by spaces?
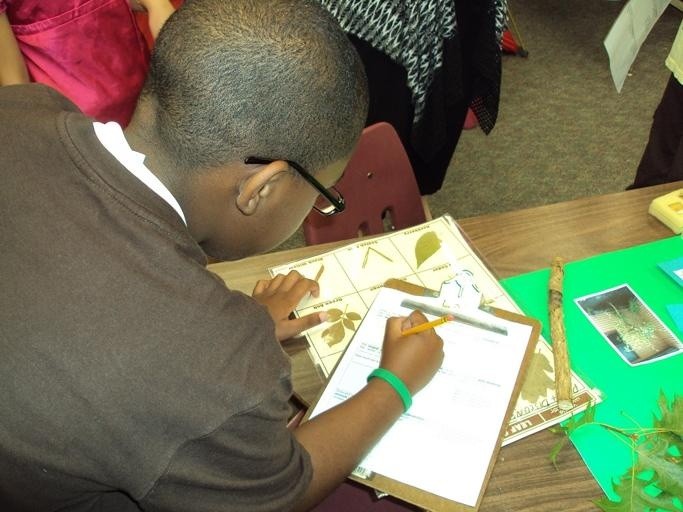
203 179 683 512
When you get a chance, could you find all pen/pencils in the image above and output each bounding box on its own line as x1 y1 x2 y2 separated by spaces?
401 315 454 336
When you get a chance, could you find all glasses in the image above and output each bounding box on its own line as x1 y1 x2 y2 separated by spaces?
244 155 344 217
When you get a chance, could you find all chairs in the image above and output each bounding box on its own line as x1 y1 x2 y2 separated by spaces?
304 122 426 246
